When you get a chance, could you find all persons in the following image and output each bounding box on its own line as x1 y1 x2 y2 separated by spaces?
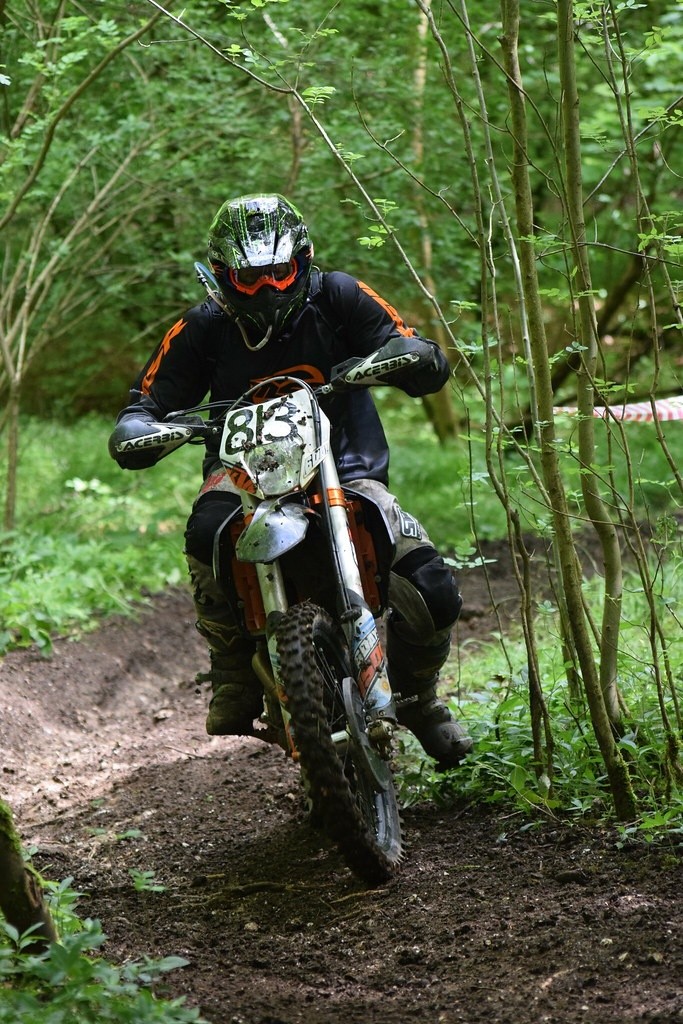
117 192 475 763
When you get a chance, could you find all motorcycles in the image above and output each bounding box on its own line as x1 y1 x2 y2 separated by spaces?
115 341 434 891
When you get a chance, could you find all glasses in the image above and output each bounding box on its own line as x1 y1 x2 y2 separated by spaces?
224 253 305 298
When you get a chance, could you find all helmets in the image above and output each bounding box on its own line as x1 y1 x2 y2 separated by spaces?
207 193 314 336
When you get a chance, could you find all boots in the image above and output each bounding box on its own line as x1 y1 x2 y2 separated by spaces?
194 598 249 735
385 632 473 760
